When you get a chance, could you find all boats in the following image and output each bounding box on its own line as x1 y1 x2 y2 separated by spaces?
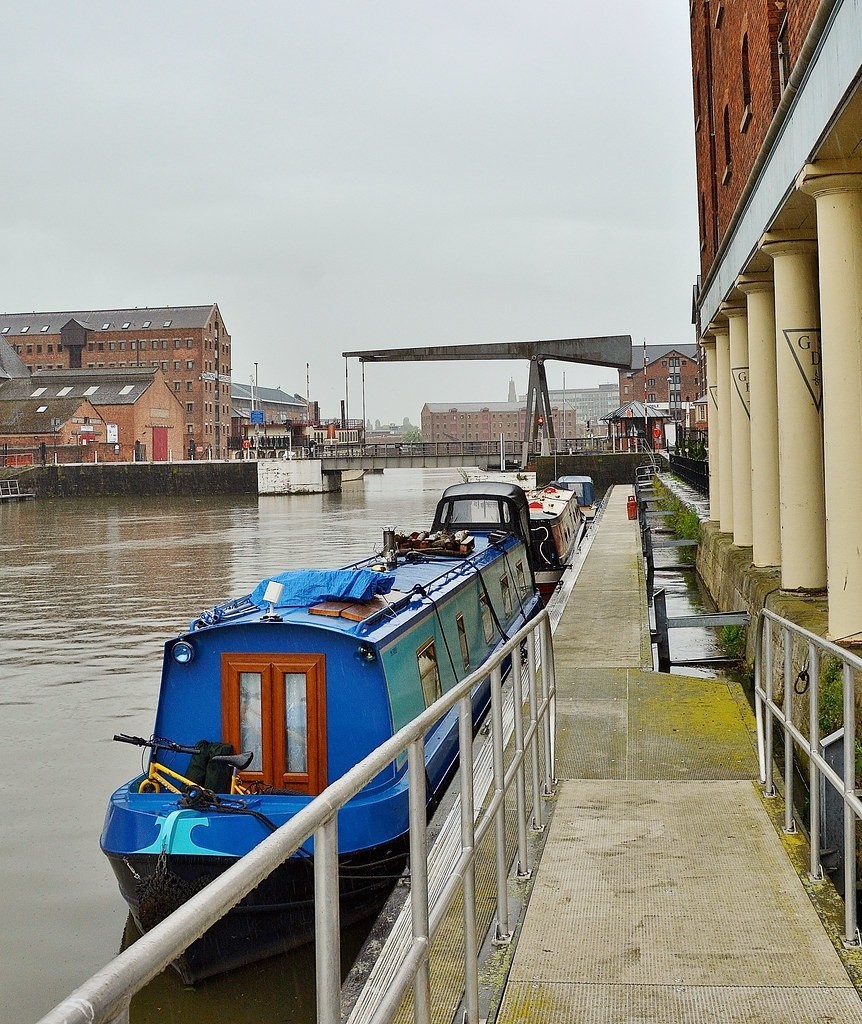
98 531 548 986
428 481 587 598
0 480 35 502
557 477 599 518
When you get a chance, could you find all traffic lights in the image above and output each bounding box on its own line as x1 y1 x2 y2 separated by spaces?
537 417 543 426
586 421 589 428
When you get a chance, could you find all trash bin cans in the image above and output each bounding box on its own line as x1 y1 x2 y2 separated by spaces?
395 443 403 455
309 440 318 456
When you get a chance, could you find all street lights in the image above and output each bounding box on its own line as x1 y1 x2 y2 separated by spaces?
667 377 672 414
254 362 258 411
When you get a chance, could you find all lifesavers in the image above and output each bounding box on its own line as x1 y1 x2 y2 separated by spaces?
544 486 555 493
242 441 250 449
